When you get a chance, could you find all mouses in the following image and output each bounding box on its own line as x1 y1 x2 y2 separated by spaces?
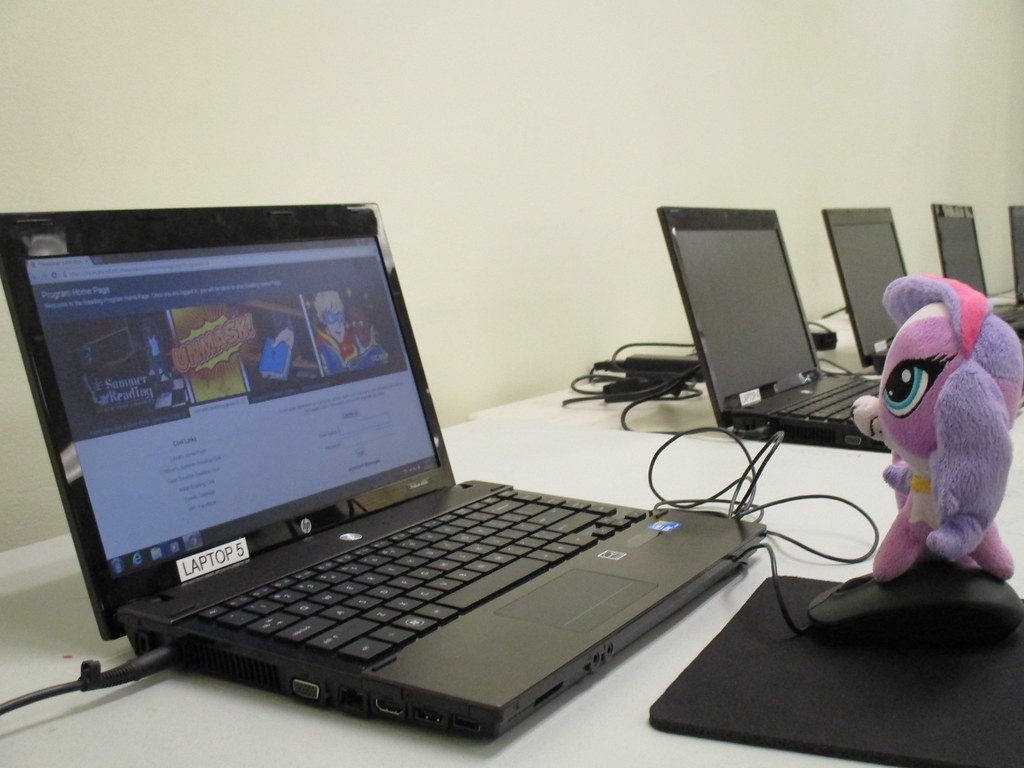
807 558 1024 647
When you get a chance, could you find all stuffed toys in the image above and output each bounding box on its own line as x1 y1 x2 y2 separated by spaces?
848 273 1023 581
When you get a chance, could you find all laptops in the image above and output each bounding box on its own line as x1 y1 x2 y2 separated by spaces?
0 201 769 744
656 204 1024 454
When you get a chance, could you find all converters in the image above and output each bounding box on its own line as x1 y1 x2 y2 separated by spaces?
623 355 704 384
809 329 837 351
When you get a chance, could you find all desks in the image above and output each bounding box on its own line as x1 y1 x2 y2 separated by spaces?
0 325 1024 768
